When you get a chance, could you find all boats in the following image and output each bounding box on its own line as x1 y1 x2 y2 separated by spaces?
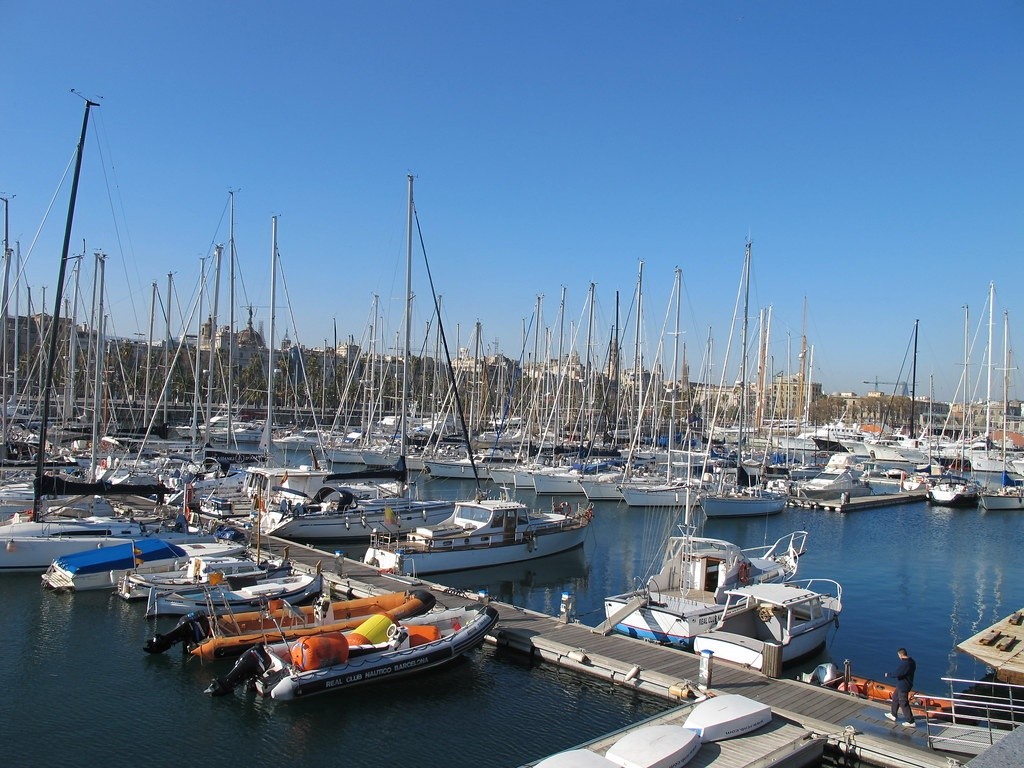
143 560 325 619
116 540 295 601
203 602 500 704
143 589 439 658
40 537 252 592
800 661 952 716
691 576 845 671
602 526 811 648
364 485 596 576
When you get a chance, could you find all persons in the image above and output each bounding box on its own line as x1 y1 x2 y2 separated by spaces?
884 649 916 728
303 503 311 514
155 480 165 505
280 497 292 513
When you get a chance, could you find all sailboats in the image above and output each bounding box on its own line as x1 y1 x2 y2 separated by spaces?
0 85 1024 571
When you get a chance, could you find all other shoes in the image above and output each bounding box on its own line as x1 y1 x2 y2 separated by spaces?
902 722 916 727
884 713 896 721
